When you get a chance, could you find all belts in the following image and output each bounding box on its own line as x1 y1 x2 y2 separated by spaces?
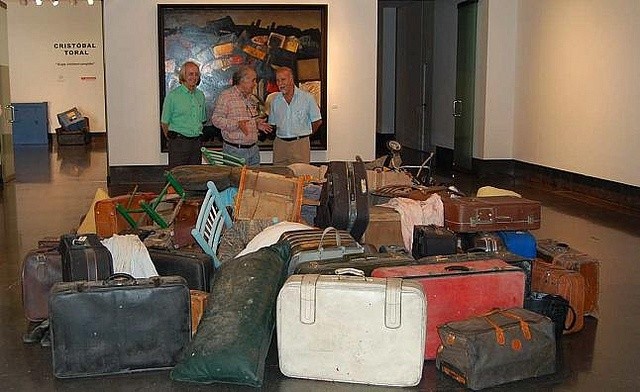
224 140 259 149
178 134 204 141
278 135 311 141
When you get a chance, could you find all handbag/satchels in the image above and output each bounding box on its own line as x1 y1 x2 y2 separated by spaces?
367 168 415 198
524 290 579 346
435 305 560 392
56 107 92 145
474 171 520 200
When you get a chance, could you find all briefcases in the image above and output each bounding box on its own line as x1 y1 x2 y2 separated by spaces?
443 195 544 232
533 259 585 335
538 237 600 319
293 249 416 276
370 259 529 361
274 271 427 389
47 272 193 379
144 244 215 294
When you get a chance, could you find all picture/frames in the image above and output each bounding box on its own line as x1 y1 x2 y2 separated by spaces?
157 3 328 153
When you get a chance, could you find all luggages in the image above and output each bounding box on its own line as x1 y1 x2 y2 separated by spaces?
412 225 458 258
463 235 504 256
21 248 63 324
60 234 114 284
277 228 360 254
501 231 536 262
94 189 154 241
315 158 370 240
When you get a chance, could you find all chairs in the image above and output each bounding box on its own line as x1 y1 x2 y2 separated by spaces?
190 180 232 270
201 146 246 168
233 166 305 223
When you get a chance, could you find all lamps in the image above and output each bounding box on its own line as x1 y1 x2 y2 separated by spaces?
50 0 59 6
69 0 76 6
88 0 94 6
19 0 27 6
34 0 43 6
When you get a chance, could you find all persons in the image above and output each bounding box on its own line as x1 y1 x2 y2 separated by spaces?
267 67 323 167
211 65 273 168
160 62 209 169
